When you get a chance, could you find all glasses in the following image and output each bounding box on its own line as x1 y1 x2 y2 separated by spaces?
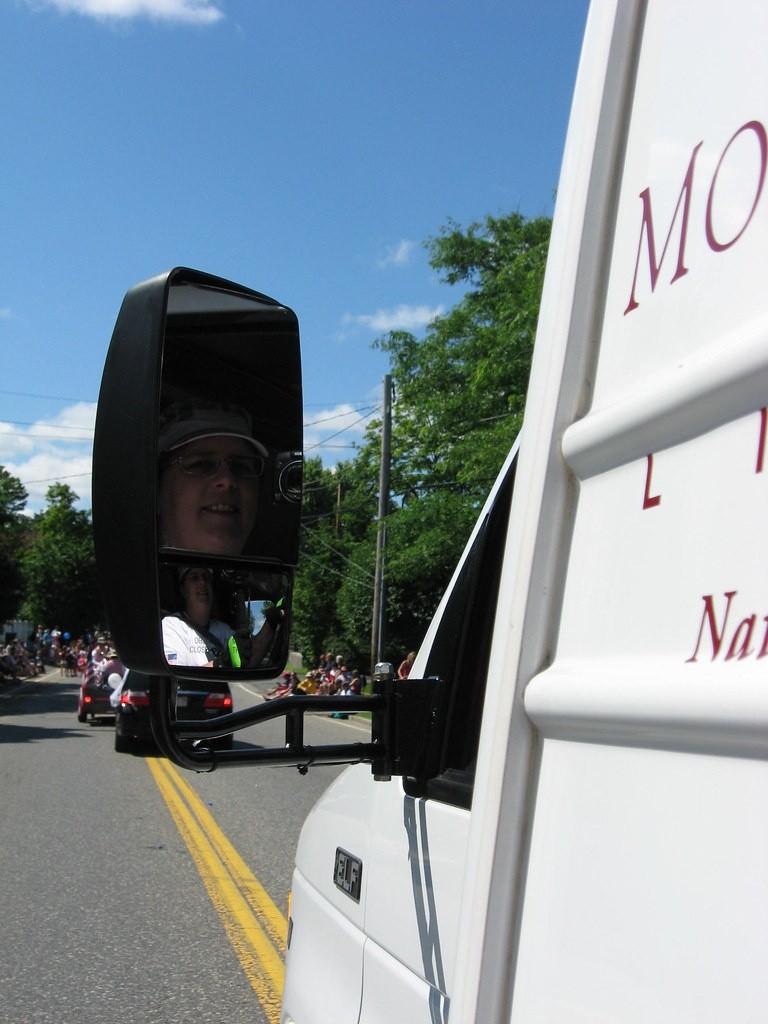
161 453 264 479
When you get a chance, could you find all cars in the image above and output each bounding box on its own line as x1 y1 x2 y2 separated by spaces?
115 668 234 752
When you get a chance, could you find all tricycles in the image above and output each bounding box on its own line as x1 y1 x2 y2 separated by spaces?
78 664 117 721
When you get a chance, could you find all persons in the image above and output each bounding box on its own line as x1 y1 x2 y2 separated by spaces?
398 652 415 680
160 565 284 670
0 625 124 689
156 391 269 558
263 653 361 719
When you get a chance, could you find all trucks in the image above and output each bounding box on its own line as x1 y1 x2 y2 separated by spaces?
92 0 768 1024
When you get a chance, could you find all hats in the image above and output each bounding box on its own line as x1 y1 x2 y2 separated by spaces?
96 637 107 644
305 672 312 678
282 671 290 678
341 667 347 672
104 650 117 658
156 395 269 459
